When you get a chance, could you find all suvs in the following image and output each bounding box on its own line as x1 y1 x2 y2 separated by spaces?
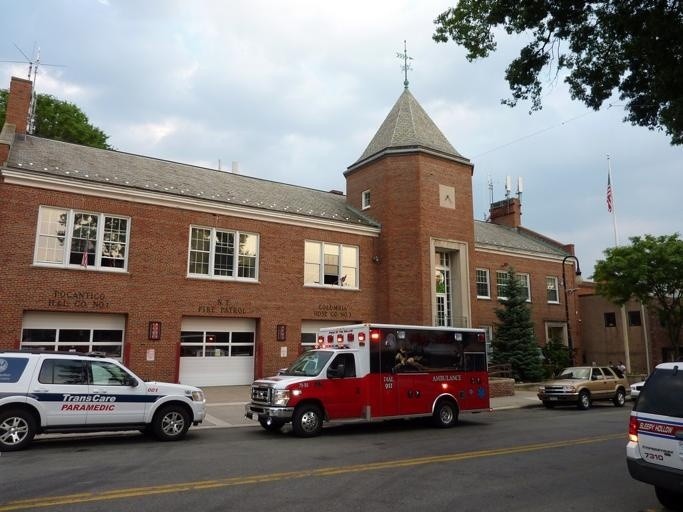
0 348 205 448
536 364 630 409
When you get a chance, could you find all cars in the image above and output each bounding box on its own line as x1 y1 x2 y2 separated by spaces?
629 380 645 400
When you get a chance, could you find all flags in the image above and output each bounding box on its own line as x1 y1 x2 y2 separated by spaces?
79 233 90 267
605 165 612 214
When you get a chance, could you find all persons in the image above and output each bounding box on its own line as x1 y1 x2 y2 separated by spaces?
616 360 625 373
607 360 613 366
591 358 599 367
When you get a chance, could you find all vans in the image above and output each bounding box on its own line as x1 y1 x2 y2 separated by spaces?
622 362 682 512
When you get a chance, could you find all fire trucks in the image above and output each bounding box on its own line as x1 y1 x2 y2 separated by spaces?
244 323 492 436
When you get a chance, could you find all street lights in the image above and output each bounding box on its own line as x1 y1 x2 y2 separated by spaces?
561 254 584 364
